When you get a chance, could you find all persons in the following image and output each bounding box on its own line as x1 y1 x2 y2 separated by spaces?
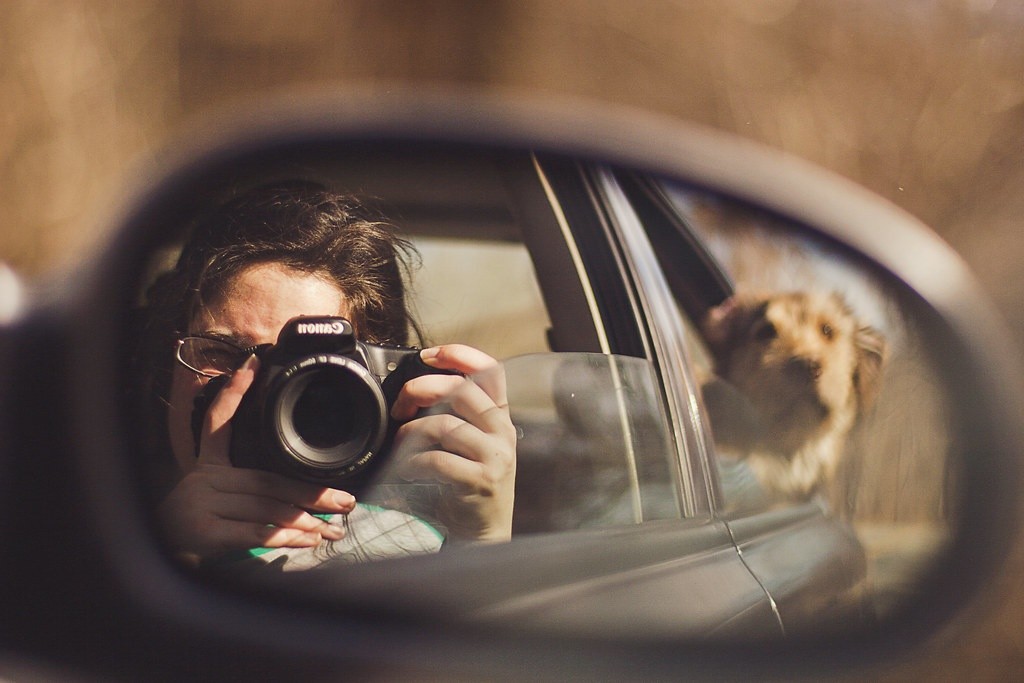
153 176 519 586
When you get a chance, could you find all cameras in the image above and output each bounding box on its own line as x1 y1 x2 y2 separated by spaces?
189 313 467 490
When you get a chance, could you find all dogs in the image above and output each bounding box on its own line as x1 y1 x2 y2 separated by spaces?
693 287 888 515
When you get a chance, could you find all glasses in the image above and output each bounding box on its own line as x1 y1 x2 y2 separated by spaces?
171 323 247 381
